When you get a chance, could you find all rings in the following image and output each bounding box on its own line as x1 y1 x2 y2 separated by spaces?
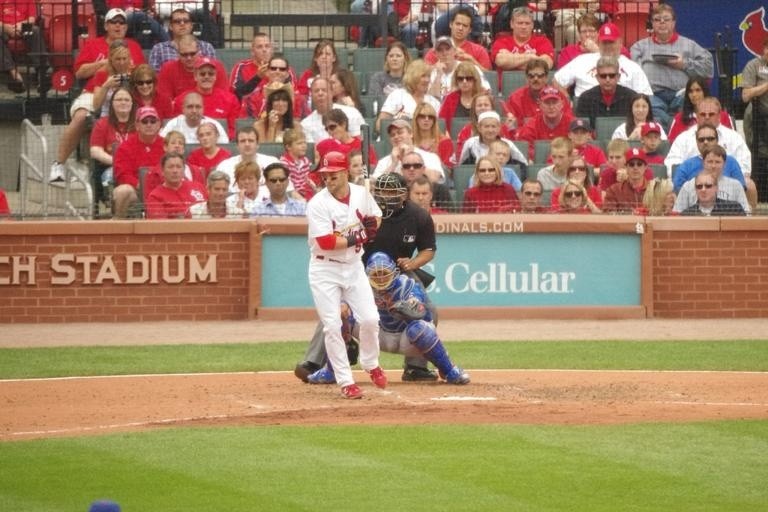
405 263 409 268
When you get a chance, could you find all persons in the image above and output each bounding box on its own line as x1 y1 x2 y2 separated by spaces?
303 151 388 402
2 0 767 219
305 249 473 386
293 171 438 385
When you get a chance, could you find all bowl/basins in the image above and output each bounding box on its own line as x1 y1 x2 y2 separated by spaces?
653 55 677 64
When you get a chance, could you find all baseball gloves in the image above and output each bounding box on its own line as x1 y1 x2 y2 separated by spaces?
389 297 427 320
642 177 675 215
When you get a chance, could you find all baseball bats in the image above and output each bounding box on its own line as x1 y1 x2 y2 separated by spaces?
361 124 371 218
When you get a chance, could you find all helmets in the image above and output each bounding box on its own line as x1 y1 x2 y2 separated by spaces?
373 173 408 219
364 251 400 290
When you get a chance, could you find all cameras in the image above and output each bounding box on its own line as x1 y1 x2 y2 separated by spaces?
115 73 130 81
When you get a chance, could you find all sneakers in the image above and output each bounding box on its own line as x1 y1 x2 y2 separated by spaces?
339 384 363 399
307 367 335 384
294 362 316 383
439 367 470 384
401 366 438 381
365 366 388 389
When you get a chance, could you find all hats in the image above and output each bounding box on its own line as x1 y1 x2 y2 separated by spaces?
569 118 592 131
598 25 622 44
316 150 349 174
434 35 453 49
624 145 647 163
640 122 660 136
105 8 129 22
387 119 412 134
478 111 501 124
538 85 563 102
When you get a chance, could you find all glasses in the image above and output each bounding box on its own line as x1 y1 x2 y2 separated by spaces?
568 166 586 172
134 50 295 125
628 163 642 167
598 73 619 78
479 168 495 173
173 18 191 23
564 192 583 197
403 163 422 169
525 191 541 197
688 88 702 93
268 175 286 182
696 184 713 189
527 72 545 79
698 136 716 142
654 16 670 22
699 112 716 117
418 114 435 120
325 124 337 132
457 77 473 82
